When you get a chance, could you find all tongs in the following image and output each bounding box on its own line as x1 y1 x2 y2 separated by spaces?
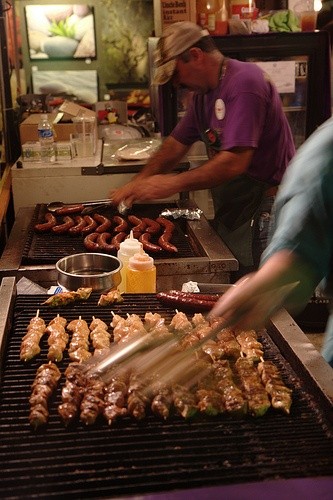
47 200 110 213
90 270 312 392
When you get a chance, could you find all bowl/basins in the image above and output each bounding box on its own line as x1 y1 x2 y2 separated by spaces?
55 252 122 291
47 96 78 122
16 95 46 109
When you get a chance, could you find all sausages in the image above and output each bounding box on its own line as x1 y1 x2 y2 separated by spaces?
35 205 179 253
156 289 222 312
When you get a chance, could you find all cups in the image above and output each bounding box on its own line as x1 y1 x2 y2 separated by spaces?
71 117 97 158
302 11 316 31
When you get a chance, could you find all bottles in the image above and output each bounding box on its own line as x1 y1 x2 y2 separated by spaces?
199 0 255 36
117 230 158 293
37 115 55 163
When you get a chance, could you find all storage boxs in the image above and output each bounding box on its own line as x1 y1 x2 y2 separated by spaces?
19 100 98 162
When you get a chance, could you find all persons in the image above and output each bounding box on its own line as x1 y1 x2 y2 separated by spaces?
211 118 333 366
109 22 296 280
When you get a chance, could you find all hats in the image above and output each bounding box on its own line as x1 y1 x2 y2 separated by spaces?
151 21 210 85
